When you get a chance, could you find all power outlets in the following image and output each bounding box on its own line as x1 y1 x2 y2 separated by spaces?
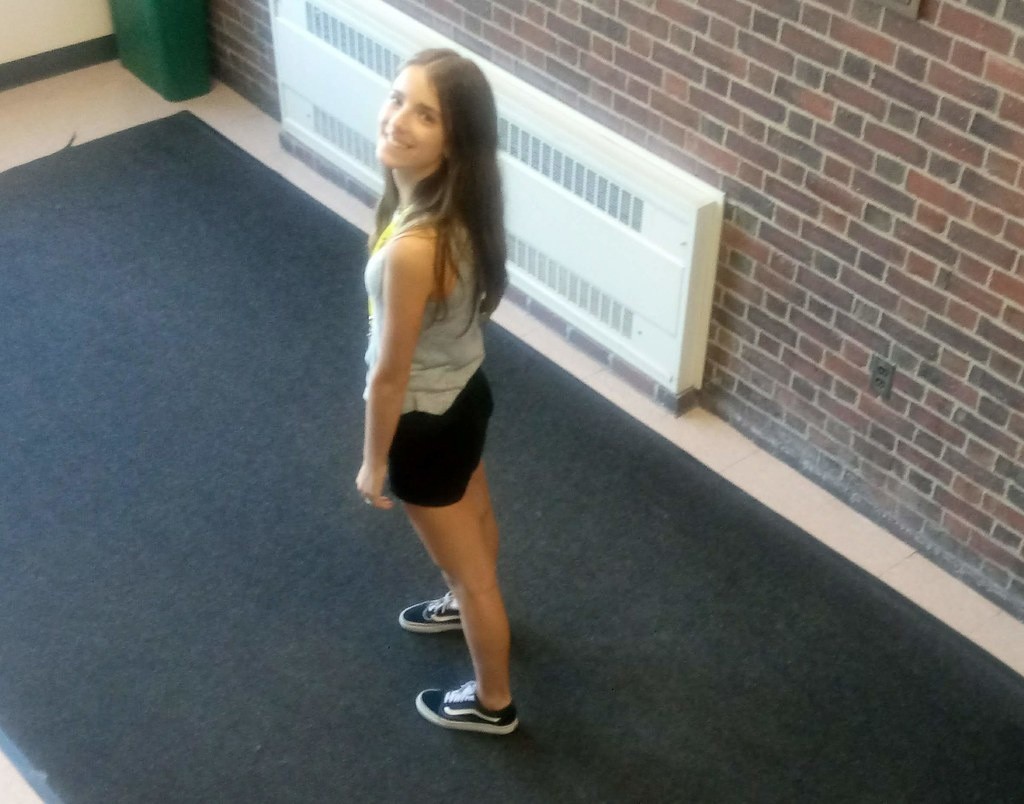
869 354 896 398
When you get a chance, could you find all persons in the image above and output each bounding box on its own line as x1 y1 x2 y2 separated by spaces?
355 48 520 733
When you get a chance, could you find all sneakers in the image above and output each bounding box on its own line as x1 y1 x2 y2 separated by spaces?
415 681 518 734
399 590 462 633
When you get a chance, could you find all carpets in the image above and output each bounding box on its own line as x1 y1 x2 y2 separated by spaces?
0 111 1023 804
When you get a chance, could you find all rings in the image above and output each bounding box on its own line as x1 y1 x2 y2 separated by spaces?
365 498 373 505
